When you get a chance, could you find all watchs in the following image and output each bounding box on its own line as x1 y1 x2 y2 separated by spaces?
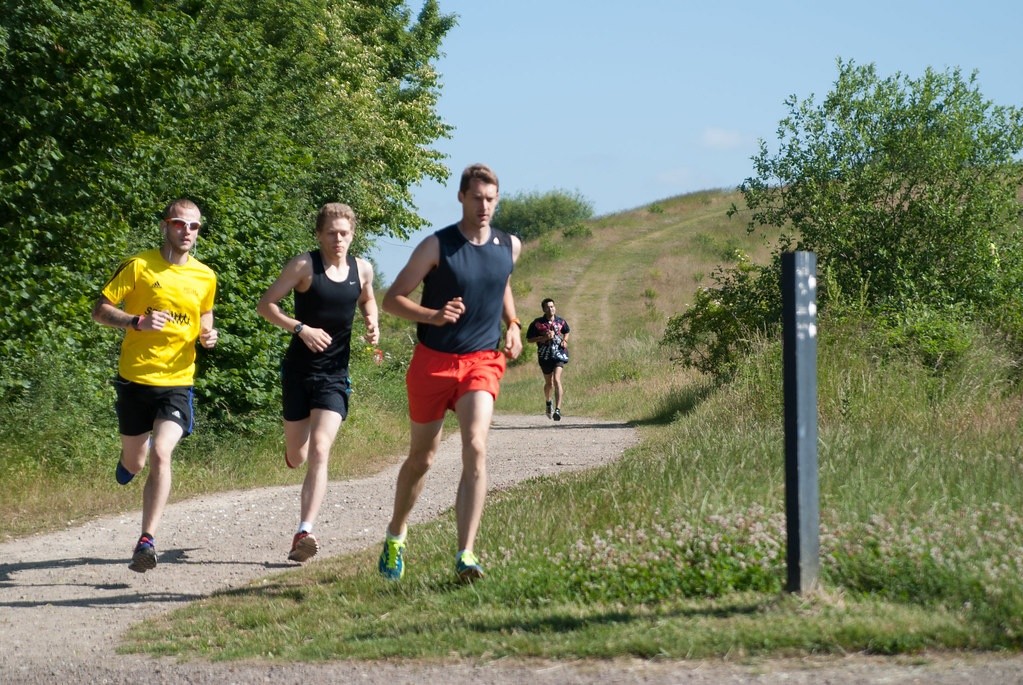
131 314 145 331
294 322 304 335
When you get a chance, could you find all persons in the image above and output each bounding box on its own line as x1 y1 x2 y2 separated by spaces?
378 163 523 580
92 199 218 574
257 203 380 562
525 298 571 421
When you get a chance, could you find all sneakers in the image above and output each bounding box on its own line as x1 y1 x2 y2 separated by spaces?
379 522 408 580
288 529 318 563
553 408 561 422
453 551 484 583
284 451 297 470
546 400 553 419
127 537 159 573
116 435 153 486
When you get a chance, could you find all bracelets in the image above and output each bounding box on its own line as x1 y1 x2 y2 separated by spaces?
506 319 522 329
564 340 567 343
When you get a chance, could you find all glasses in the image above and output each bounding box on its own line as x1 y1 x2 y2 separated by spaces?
164 218 202 231
